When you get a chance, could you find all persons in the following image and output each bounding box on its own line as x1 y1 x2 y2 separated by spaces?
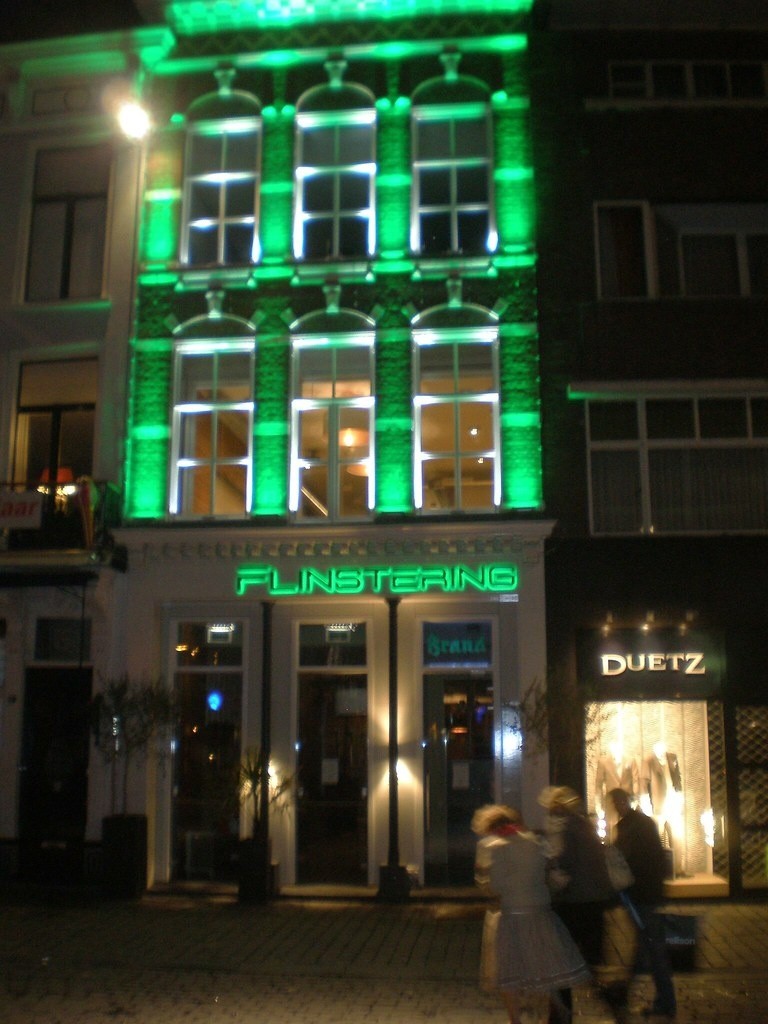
471 784 681 1024
594 736 695 880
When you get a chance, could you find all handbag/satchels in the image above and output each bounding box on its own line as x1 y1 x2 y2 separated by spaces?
634 910 698 974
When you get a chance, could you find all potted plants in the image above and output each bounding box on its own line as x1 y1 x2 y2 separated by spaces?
228 745 305 907
85 669 191 901
